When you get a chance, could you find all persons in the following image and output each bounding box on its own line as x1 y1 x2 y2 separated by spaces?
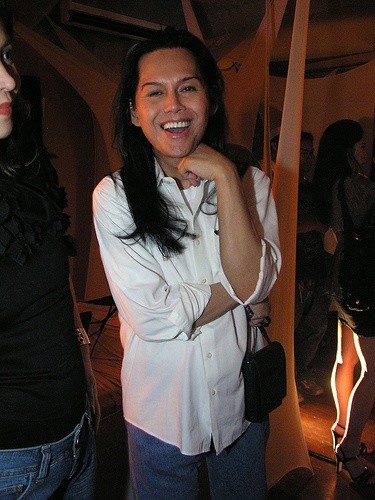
90 29 283 500
295 119 375 488
0 5 99 500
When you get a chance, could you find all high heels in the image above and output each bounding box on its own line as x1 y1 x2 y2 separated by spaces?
333 444 375 489
329 422 374 457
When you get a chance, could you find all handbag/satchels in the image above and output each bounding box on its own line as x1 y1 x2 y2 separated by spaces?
241 341 289 424
331 233 375 338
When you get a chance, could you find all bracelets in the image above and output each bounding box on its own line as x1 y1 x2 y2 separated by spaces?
75 327 91 345
